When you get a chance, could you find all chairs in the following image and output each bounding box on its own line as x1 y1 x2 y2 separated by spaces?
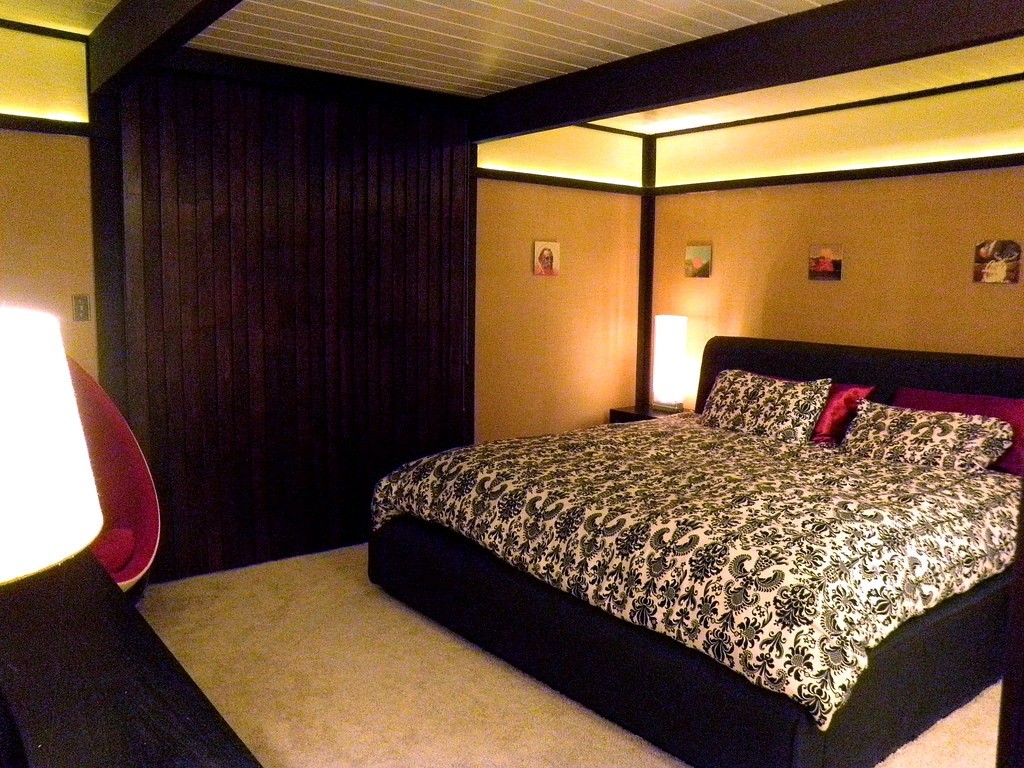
67 357 161 593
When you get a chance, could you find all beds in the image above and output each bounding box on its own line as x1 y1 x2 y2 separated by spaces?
366 335 1024 768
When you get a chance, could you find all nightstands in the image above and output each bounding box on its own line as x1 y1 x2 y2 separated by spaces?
609 405 691 424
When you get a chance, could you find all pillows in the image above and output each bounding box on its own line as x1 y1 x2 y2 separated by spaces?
766 376 875 445
700 369 833 444
842 397 1014 474
896 386 1024 476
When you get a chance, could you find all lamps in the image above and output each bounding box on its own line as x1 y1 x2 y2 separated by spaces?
651 314 688 413
0 302 105 589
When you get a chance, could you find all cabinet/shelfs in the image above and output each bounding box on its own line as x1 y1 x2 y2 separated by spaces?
0 549 262 768
